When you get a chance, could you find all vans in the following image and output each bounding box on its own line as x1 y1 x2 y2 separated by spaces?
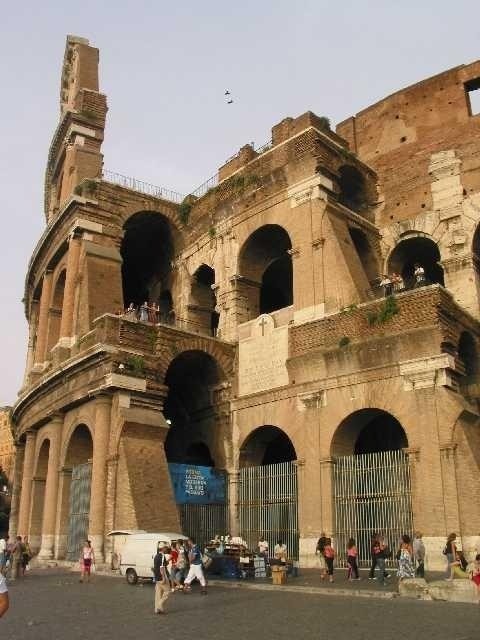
101 528 213 587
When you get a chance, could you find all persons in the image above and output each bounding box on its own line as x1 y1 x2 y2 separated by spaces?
412 263 426 283
379 274 394 296
125 301 161 324
390 270 405 290
154 541 170 615
78 540 97 583
313 531 480 589
151 533 207 595
1 534 32 620
273 540 287 562
254 534 269 557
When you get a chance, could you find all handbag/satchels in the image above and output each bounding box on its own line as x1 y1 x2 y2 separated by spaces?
416 563 424 577
373 546 383 554
443 545 448 555
201 554 213 569
397 549 401 560
472 575 480 585
